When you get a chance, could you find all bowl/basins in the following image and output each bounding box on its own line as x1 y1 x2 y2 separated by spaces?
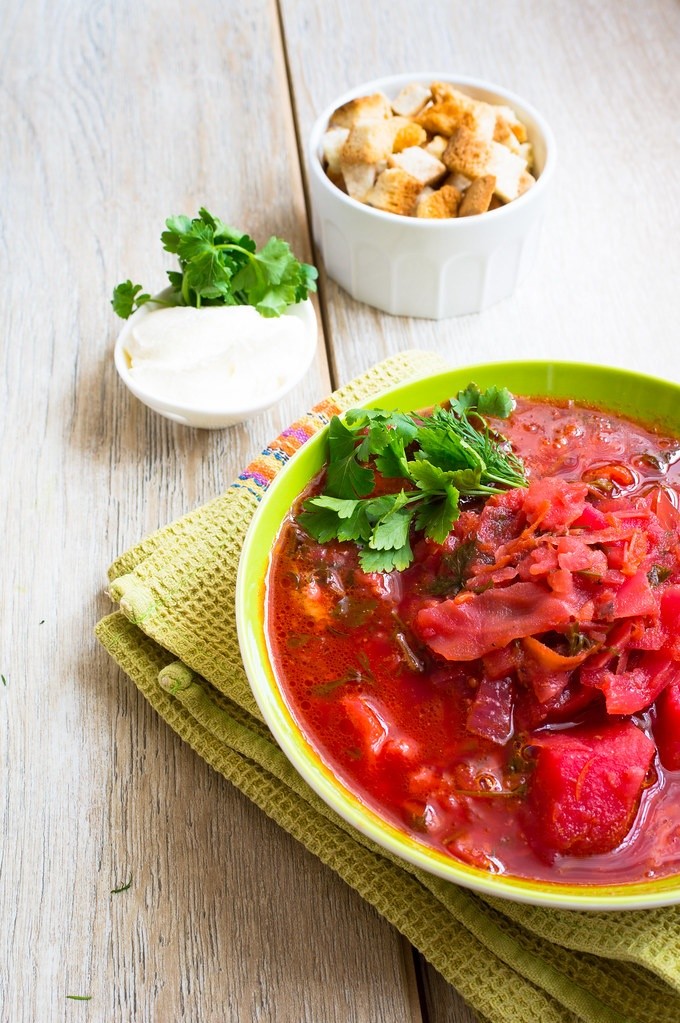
233 359 680 912
112 281 317 430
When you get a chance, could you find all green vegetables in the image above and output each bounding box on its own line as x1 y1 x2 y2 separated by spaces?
110 208 319 319
293 380 536 574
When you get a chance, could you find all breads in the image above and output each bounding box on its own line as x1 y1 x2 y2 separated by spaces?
319 83 538 219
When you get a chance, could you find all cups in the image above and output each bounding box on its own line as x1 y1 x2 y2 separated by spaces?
305 72 557 319
